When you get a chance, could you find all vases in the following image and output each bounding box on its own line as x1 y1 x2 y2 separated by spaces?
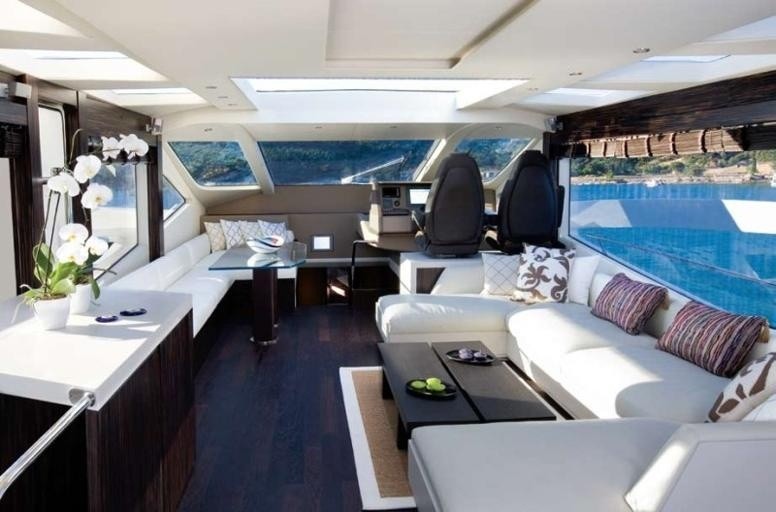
32 295 71 330
68 283 92 314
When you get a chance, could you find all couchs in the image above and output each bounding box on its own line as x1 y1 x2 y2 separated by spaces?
522 242 576 257
569 254 601 306
704 351 776 423
374 261 776 512
589 273 668 336
481 253 520 297
106 231 295 381
653 301 767 377
510 253 574 303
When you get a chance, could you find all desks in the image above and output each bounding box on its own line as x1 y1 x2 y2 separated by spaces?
208 240 308 347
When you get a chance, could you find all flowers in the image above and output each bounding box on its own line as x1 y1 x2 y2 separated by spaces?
13 134 150 300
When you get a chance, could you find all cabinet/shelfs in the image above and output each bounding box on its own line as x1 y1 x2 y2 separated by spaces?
1 309 197 512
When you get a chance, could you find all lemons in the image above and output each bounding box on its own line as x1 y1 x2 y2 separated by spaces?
410 376 447 392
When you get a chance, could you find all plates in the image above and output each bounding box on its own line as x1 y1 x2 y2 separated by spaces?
404 378 460 401
445 349 496 366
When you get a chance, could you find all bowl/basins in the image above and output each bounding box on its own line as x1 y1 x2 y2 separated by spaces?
244 234 286 254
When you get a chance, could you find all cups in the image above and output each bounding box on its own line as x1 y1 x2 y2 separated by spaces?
93 282 118 318
120 278 144 313
279 242 309 266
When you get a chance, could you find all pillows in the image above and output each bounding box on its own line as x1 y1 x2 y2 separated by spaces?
238 220 257 239
257 219 290 241
430 264 485 296
219 219 248 250
203 221 226 254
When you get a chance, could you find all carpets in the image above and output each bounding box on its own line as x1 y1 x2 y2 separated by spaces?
339 361 567 510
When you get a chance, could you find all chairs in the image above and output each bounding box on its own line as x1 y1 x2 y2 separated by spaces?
410 153 485 258
484 150 566 255
